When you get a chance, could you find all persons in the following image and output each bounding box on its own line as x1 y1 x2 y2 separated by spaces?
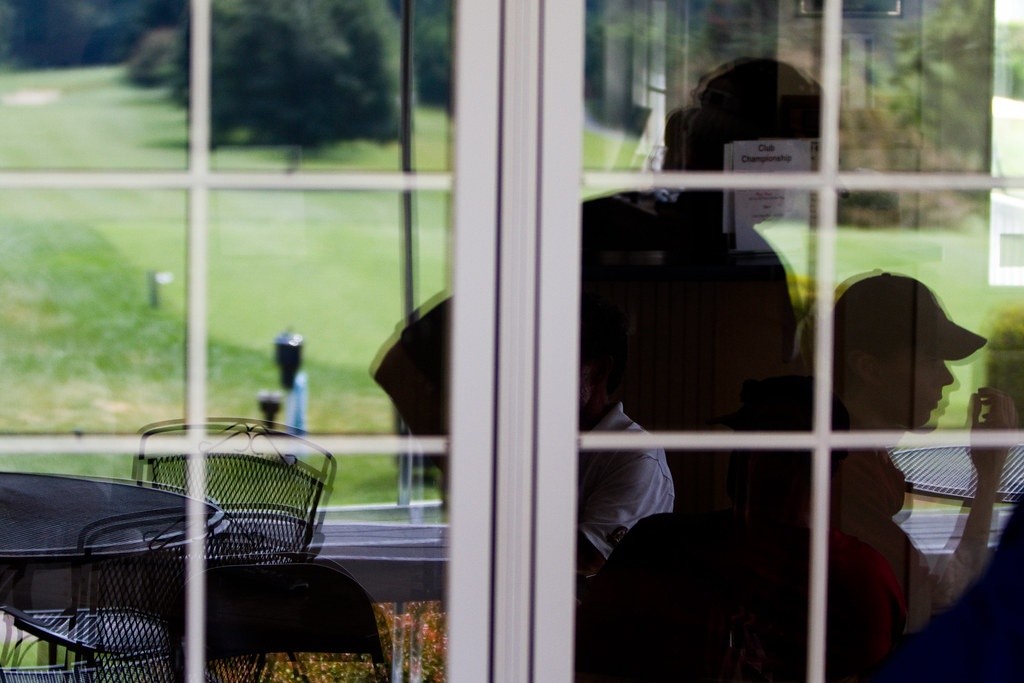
368 50 1024 682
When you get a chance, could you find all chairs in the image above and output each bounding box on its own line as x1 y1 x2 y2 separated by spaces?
0 503 314 683
171 552 389 683
14 417 338 665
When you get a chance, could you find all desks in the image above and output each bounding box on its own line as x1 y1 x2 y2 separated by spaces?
0 470 232 633
890 447 1024 502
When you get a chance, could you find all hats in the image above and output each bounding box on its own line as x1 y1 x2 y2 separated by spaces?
833 272 989 359
